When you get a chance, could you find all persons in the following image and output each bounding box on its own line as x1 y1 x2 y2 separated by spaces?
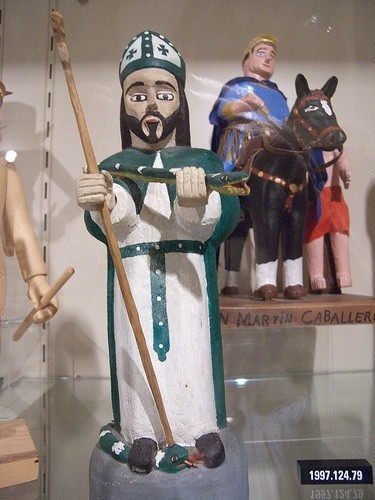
206 32 354 297
74 31 242 474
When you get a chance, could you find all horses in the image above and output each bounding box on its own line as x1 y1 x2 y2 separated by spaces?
219 71 348 304
